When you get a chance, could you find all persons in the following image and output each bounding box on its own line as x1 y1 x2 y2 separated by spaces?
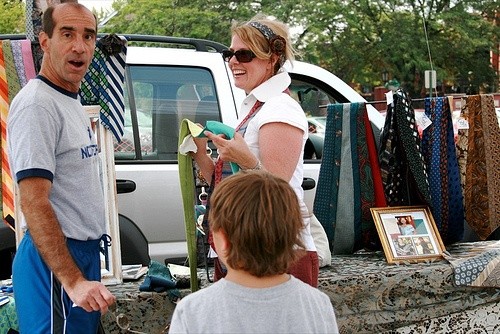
7 3 116 334
190 15 319 287
399 216 415 234
417 238 432 254
168 172 339 334
395 238 414 256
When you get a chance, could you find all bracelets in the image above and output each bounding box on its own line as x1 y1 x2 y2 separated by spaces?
243 159 261 171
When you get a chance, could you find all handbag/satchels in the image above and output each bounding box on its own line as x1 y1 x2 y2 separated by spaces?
206 87 293 287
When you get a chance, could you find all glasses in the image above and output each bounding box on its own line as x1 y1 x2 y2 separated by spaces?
221 49 256 62
108 302 145 333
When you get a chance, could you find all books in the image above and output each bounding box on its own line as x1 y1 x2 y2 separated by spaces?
122 264 149 280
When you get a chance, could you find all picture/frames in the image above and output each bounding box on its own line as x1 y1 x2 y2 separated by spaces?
12 102 123 286
368 204 446 263
